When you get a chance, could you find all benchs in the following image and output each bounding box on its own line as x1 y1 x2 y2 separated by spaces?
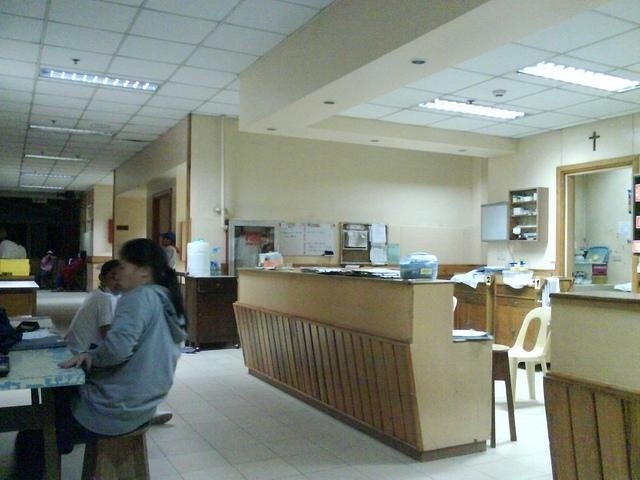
80 422 152 480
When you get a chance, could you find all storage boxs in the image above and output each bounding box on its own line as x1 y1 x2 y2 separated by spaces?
502 270 533 286
399 252 441 279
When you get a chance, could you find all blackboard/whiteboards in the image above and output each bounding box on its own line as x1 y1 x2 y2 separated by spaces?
280 222 335 258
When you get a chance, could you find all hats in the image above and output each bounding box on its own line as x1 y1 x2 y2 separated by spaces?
160 232 175 240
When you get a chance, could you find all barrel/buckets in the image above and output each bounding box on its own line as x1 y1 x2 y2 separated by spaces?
186 241 210 276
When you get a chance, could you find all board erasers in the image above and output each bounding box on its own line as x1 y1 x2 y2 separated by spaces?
326 251 334 255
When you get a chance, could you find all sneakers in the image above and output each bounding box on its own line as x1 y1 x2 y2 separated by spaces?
150 410 172 425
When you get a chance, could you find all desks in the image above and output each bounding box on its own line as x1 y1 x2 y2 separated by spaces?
0 280 40 318
0 316 86 480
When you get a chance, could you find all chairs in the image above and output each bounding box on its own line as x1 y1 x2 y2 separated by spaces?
509 305 551 405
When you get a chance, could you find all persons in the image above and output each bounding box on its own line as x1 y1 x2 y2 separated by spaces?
52 251 85 291
159 230 177 272
36 250 57 289
9 238 188 480
64 259 174 424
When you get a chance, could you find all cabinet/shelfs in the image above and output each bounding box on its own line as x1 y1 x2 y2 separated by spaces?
184 275 239 352
575 261 614 289
340 222 388 266
509 187 549 244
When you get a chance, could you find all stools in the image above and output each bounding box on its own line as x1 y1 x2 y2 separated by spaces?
491 344 517 448
